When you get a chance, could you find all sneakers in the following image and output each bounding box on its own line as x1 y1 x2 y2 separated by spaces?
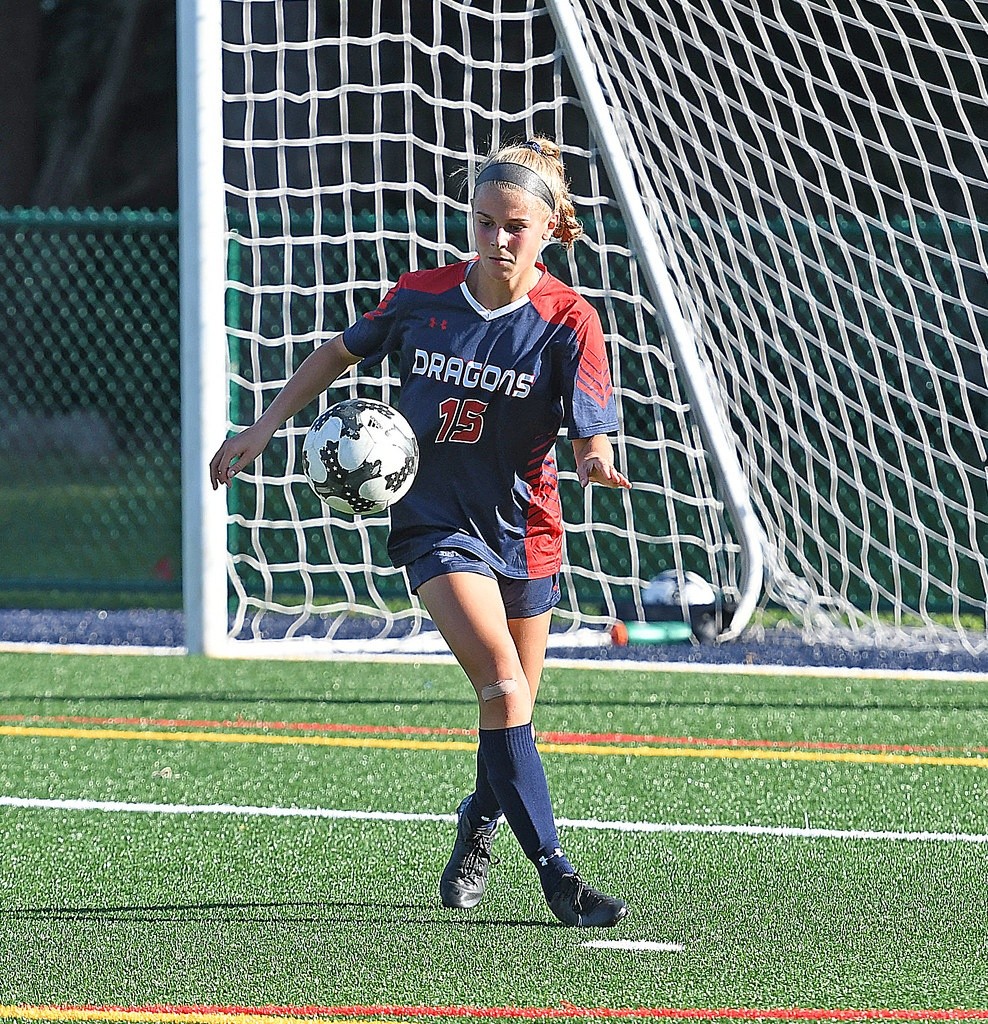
547 867 627 927
440 796 499 910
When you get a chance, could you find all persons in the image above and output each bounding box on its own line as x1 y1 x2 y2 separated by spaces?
209 137 632 924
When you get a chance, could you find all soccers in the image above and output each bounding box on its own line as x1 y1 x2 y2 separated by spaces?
298 396 420 517
